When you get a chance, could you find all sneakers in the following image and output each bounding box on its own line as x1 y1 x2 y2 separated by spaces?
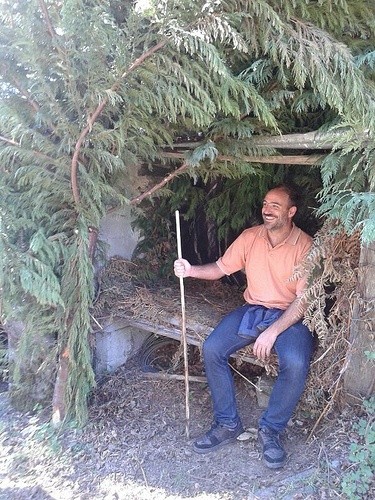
192 420 243 454
257 424 286 469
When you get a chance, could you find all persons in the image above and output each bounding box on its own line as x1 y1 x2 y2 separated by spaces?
173 186 324 469
